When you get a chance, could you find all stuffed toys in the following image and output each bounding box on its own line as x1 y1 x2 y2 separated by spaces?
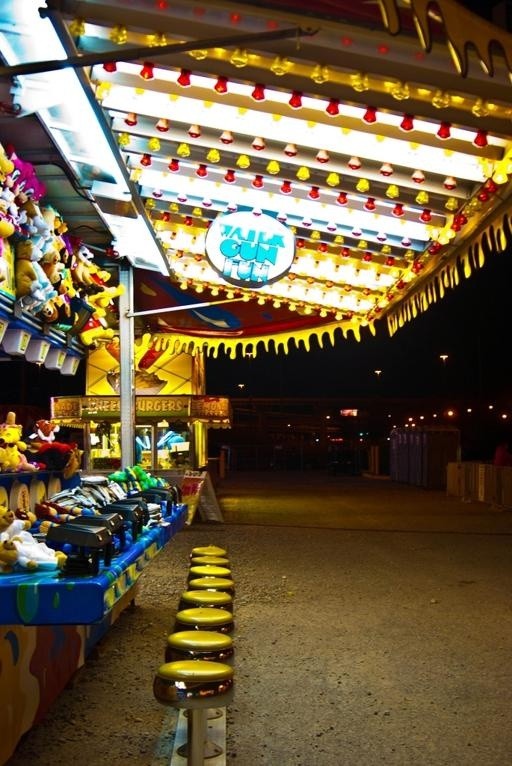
1 411 38 472
0 461 179 578
1 139 127 348
31 418 79 471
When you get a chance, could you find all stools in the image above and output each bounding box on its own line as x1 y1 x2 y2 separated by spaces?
153 545 235 766
